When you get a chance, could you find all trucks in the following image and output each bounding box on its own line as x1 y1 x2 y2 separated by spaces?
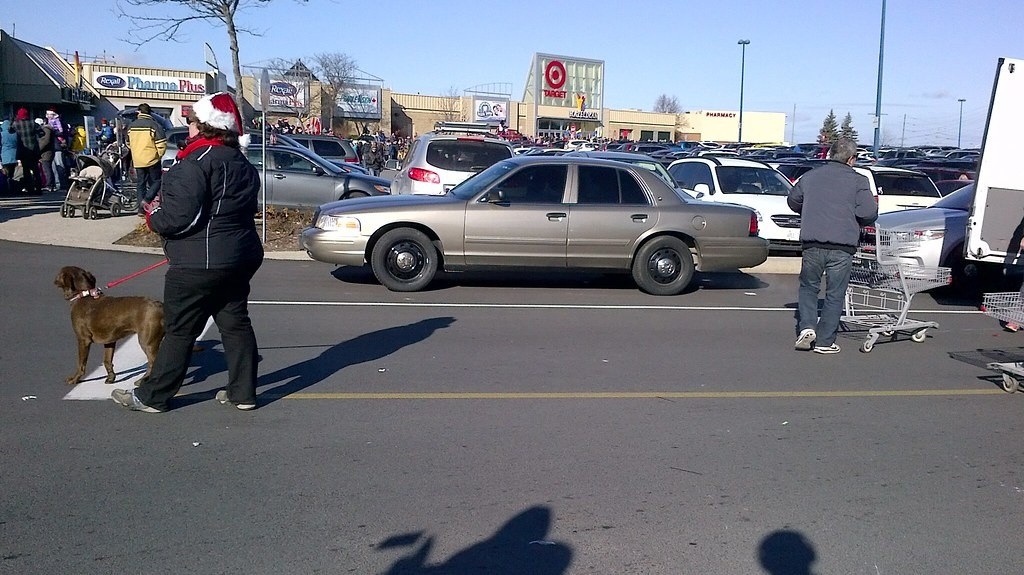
496 129 523 142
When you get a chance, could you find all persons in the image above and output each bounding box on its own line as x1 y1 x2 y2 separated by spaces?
126 103 167 217
498 120 673 144
110 91 264 413
787 137 878 354
0 108 113 195
243 115 418 177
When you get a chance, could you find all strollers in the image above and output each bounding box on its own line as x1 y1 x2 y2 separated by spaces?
60 143 139 220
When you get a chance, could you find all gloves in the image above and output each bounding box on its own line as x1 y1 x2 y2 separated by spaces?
144 195 160 232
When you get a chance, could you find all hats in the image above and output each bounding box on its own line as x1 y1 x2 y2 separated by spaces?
372 144 376 148
192 92 250 147
277 117 311 129
46 108 59 119
17 108 28 119
102 118 114 124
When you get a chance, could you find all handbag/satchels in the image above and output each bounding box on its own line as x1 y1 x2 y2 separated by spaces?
57 136 67 148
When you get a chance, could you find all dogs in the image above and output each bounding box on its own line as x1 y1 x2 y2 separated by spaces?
55 266 206 385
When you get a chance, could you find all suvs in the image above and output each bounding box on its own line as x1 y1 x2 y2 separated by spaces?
661 155 803 256
386 120 514 196
284 135 360 166
762 159 942 243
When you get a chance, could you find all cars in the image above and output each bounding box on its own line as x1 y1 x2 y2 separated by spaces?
859 180 979 300
670 139 981 181
161 129 392 210
301 157 771 296
509 140 671 177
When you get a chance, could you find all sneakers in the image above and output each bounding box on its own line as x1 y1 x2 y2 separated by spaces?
216 390 256 411
1005 322 1019 331
794 329 816 349
813 343 841 353
111 389 161 413
40 187 58 192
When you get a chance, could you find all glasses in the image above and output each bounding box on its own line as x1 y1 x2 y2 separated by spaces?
186 117 194 125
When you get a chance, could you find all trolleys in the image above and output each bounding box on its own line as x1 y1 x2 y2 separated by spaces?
839 220 952 353
980 287 1024 393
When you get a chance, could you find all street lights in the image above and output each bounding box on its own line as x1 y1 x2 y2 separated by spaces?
738 40 750 142
958 99 966 148
